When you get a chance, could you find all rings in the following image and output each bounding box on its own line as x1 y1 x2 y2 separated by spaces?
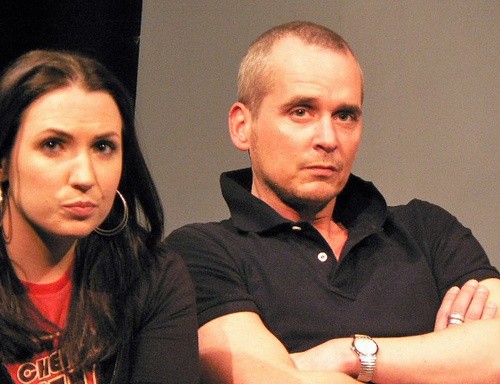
446 315 465 326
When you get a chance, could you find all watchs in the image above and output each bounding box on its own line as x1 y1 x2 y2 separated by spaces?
351 334 379 383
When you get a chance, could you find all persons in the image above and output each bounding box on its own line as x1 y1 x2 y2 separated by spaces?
161 20 500 384
0 50 199 382
